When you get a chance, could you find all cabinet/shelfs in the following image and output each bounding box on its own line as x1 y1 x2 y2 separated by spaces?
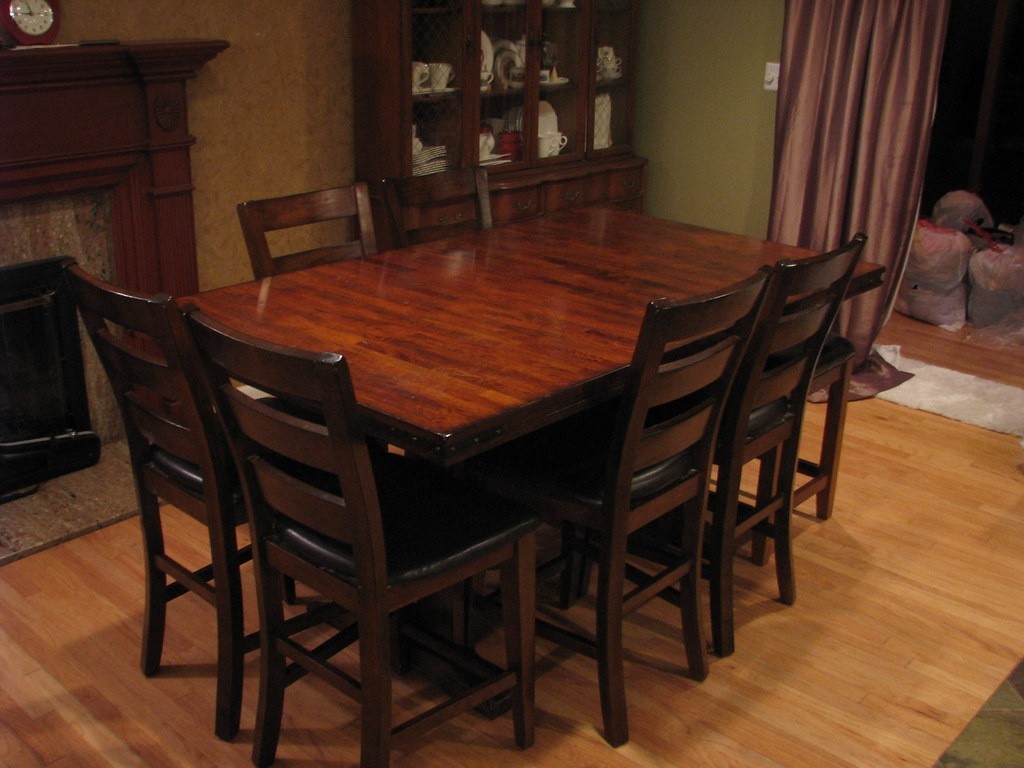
349 0 649 252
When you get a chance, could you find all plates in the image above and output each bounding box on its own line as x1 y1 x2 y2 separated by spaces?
544 77 568 84
412 144 447 177
503 100 558 142
480 154 511 166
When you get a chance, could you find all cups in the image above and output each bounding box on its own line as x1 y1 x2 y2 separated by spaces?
412 62 429 93
480 71 493 91
538 131 568 158
509 68 524 88
541 70 549 82
598 47 622 78
480 133 493 159
429 63 454 91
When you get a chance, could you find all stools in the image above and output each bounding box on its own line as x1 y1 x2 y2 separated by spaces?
706 330 856 568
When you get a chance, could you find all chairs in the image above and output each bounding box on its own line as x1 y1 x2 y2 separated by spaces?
608 230 869 659
443 265 777 750
377 167 493 248
58 259 390 741
180 304 541 768
236 181 375 279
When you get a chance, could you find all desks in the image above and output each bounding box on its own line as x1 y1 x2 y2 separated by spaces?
174 205 886 722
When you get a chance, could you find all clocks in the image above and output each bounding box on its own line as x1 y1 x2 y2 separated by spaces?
0 0 61 46
